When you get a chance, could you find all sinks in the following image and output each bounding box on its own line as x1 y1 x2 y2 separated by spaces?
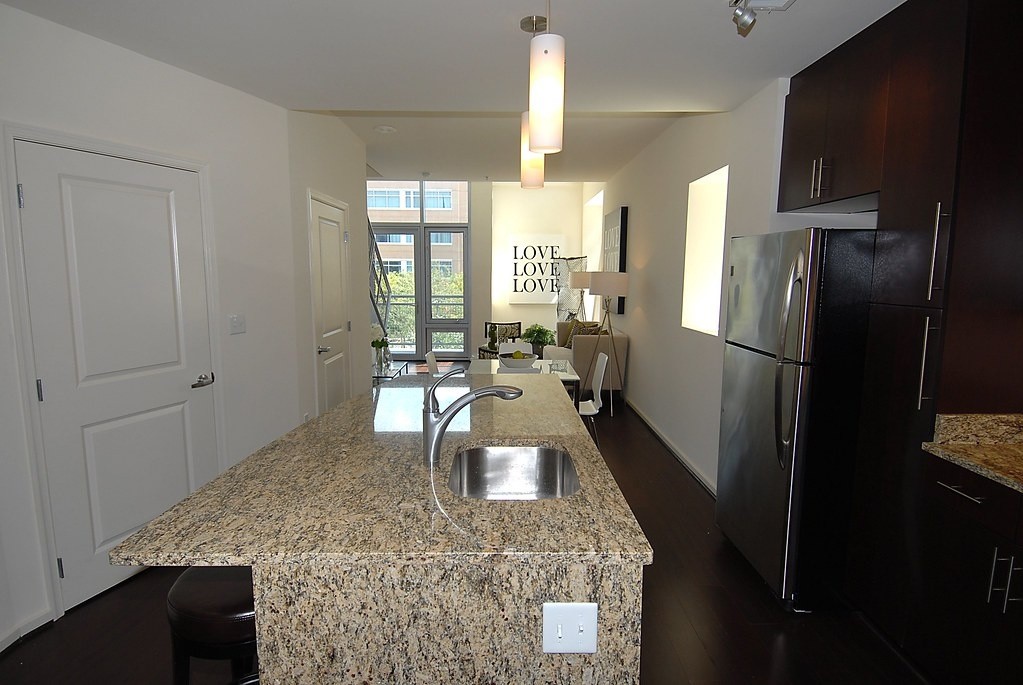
447 447 581 501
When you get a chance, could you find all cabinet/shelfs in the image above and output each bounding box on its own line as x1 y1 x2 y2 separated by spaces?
777 0 1022 685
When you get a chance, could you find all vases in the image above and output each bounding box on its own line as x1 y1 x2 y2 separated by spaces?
376 347 392 373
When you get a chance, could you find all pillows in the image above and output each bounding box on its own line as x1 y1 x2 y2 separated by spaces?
565 319 609 349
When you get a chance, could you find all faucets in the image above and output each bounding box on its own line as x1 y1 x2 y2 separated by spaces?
423 368 523 468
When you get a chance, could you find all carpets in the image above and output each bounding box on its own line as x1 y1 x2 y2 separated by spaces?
410 361 454 373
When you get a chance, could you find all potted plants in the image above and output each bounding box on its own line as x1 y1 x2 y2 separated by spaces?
520 324 557 360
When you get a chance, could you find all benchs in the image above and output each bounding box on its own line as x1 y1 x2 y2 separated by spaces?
557 320 628 401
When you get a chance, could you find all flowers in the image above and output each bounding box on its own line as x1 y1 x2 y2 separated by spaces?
370 323 390 349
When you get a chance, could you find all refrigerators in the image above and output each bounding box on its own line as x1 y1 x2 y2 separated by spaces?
714 229 876 611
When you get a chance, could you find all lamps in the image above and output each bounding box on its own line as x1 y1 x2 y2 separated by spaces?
734 7 758 30
521 111 544 188
529 0 565 153
568 270 629 408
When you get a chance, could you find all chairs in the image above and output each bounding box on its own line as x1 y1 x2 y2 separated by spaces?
544 345 574 364
566 355 610 450
425 351 466 378
498 343 532 354
479 322 521 360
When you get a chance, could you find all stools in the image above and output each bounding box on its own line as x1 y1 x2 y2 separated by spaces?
166 567 260 685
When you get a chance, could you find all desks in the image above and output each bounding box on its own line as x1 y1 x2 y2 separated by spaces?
372 362 409 386
468 358 581 415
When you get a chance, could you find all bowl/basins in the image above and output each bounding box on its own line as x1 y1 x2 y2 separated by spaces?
498 353 539 368
